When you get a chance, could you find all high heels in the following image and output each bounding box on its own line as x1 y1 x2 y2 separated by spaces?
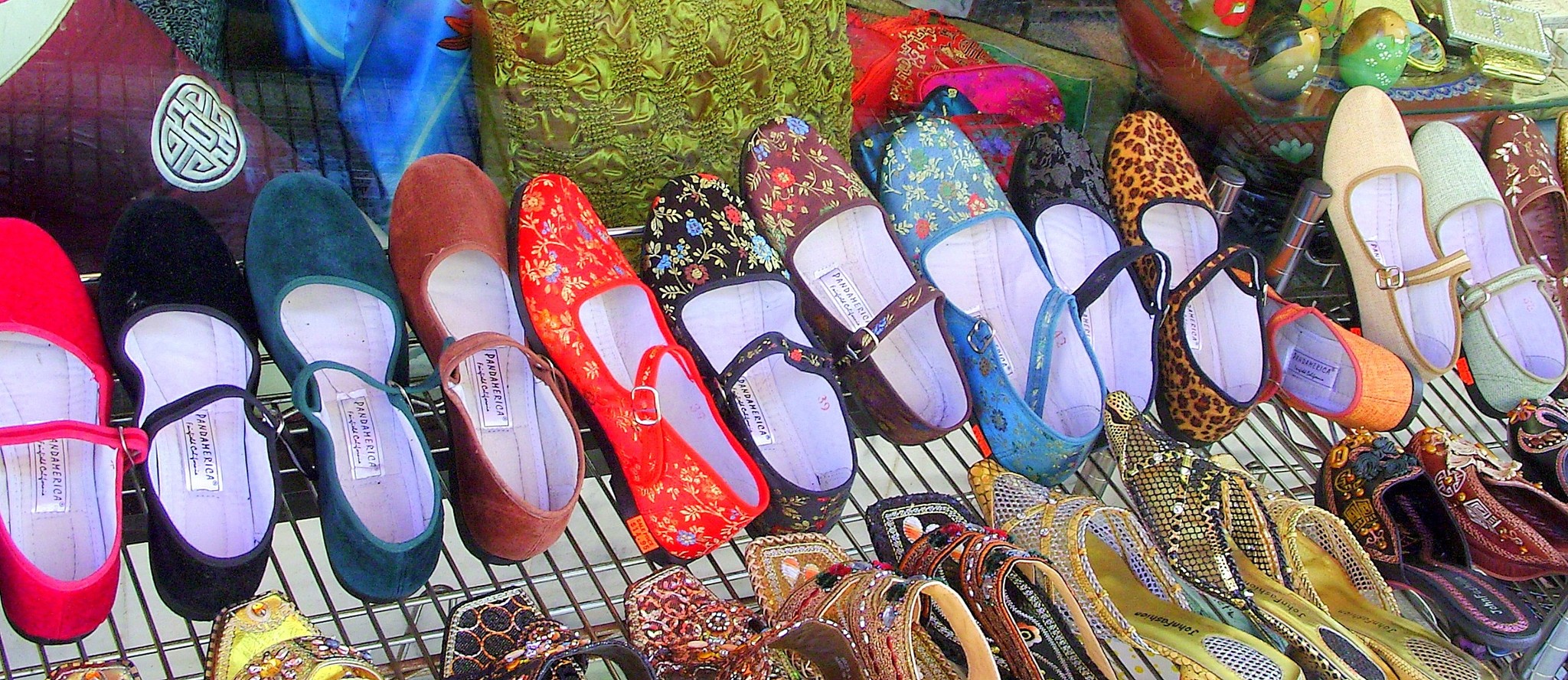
967 456 1304 680
1509 396 1568 502
1406 428 1568 587
1315 426 1535 651
1099 394 1502 679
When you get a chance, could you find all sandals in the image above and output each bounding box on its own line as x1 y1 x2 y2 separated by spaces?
868 492 1117 680
625 566 864 680
745 534 1001 679
204 586 386 680
439 586 656 680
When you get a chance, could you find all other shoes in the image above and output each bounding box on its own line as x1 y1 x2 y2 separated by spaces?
245 168 447 603
507 164 772 569
1484 111 1568 321
1011 117 1171 426
1324 87 1473 383
98 197 284 616
734 117 973 444
636 171 857 538
1412 119 1567 418
391 151 585 567
879 117 1109 486
2 215 151 649
1229 266 1421 433
1100 106 1268 447
